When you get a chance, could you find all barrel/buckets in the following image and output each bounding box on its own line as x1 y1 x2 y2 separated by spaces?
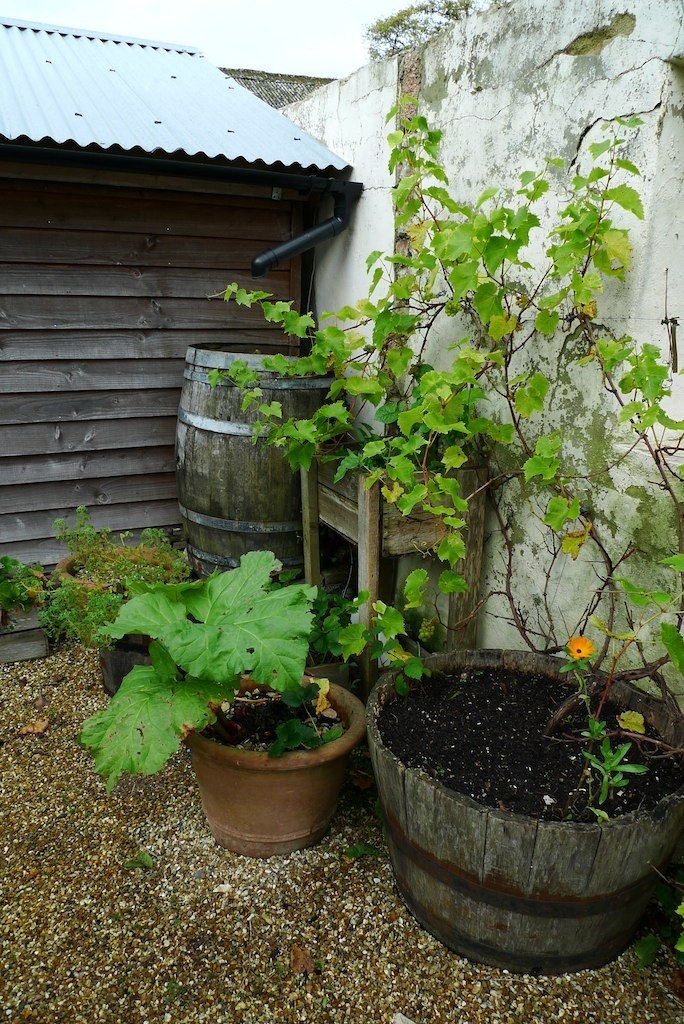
173 340 344 581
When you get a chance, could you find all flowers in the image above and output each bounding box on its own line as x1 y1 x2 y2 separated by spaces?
564 633 646 817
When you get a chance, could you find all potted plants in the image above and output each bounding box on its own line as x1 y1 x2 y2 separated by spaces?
1 104 684 969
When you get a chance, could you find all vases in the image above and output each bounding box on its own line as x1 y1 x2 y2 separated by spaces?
365 649 683 978
